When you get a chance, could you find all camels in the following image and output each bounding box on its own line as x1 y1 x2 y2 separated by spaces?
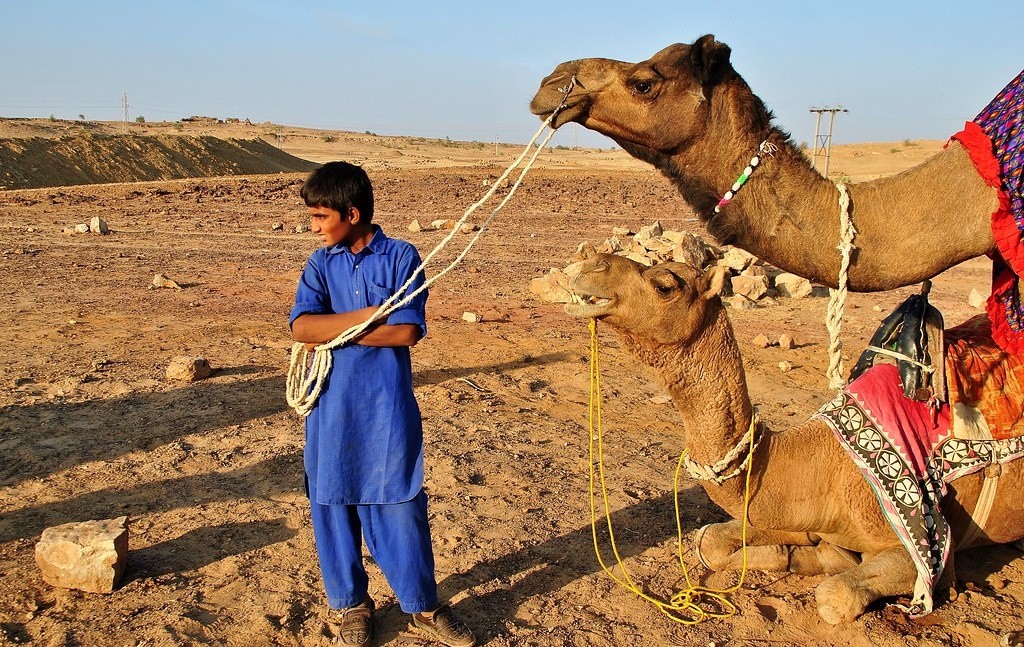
526 31 1024 344
564 251 1024 625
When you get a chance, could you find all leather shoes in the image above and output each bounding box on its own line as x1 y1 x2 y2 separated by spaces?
413 603 476 647
337 591 376 647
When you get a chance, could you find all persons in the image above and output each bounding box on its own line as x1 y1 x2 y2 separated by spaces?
287 160 478 647
